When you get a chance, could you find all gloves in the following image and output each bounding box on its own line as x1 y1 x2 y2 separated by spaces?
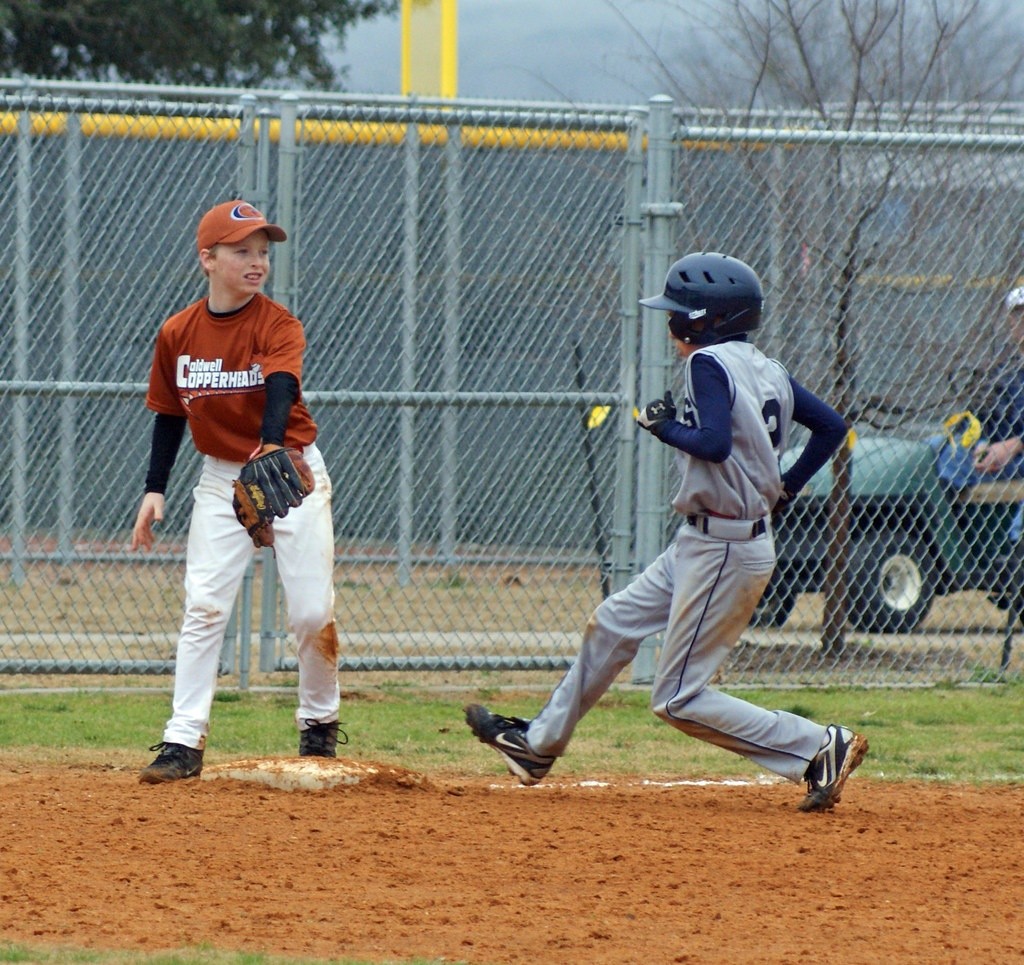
637 391 675 431
772 477 795 512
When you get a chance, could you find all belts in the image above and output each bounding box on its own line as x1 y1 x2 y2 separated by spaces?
688 514 765 541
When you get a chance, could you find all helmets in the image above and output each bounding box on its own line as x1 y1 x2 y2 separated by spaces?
637 252 764 345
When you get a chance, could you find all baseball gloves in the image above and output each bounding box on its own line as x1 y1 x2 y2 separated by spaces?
232 446 316 561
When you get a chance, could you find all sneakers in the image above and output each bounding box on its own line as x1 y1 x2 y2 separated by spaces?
465 703 557 787
800 724 869 811
299 718 347 756
139 742 204 782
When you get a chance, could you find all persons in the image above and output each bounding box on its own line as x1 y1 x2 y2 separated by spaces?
129 199 348 786
960 285 1024 607
465 252 869 812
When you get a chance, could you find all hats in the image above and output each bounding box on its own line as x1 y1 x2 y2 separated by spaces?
1006 286 1024 308
197 201 287 250
